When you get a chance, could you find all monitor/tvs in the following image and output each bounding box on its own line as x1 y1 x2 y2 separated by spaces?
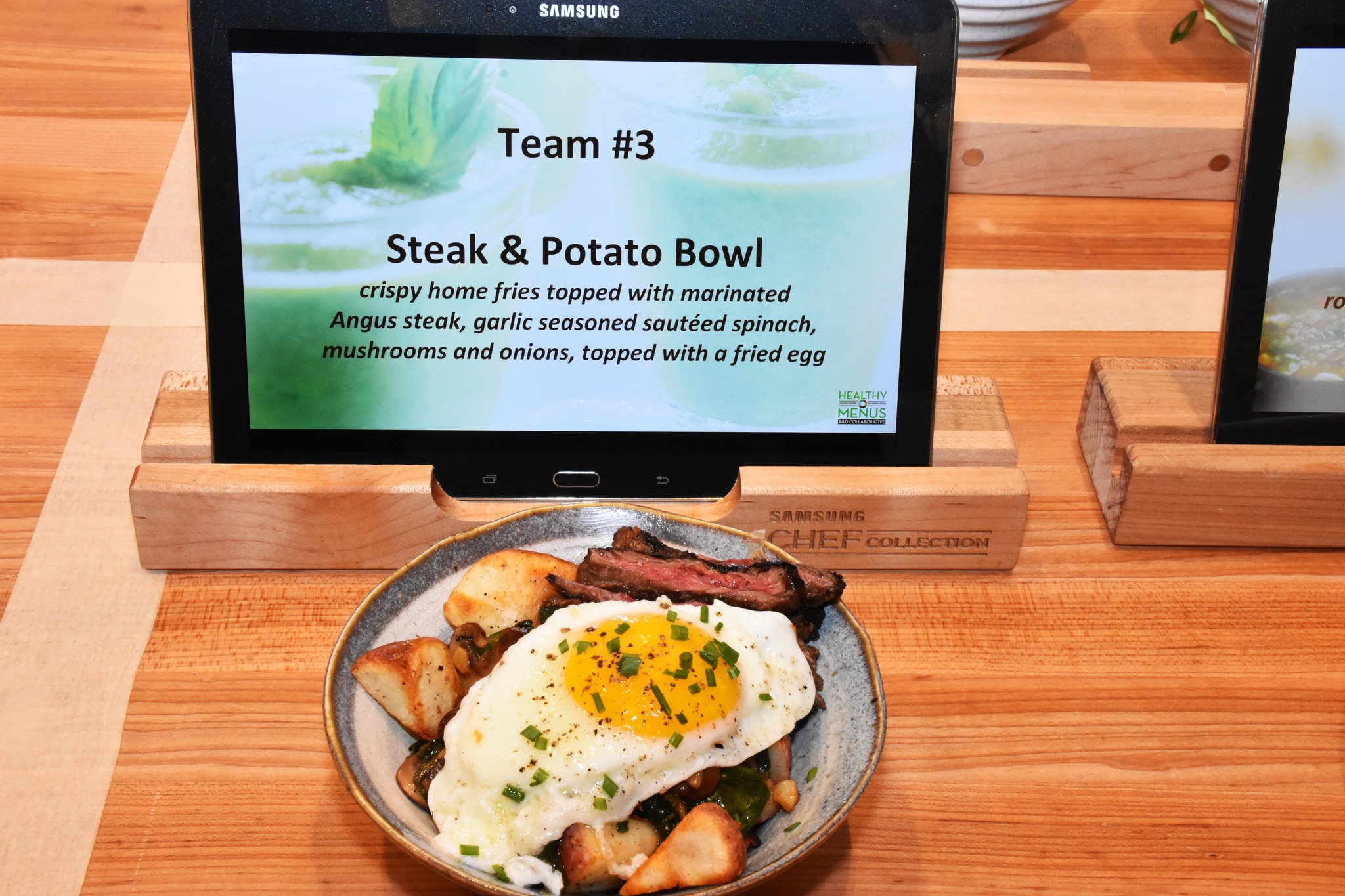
185 0 962 495
1217 0 1343 450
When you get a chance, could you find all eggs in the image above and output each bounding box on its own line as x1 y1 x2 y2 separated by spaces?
426 594 817 894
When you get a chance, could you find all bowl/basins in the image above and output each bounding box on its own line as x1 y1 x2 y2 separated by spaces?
955 0 1078 60
1201 0 1259 54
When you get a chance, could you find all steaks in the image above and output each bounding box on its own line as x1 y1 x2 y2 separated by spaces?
544 525 846 824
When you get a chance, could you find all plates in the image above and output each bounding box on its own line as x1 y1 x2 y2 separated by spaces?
324 501 887 896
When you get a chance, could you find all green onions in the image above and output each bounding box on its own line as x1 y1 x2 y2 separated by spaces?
458 603 774 885
